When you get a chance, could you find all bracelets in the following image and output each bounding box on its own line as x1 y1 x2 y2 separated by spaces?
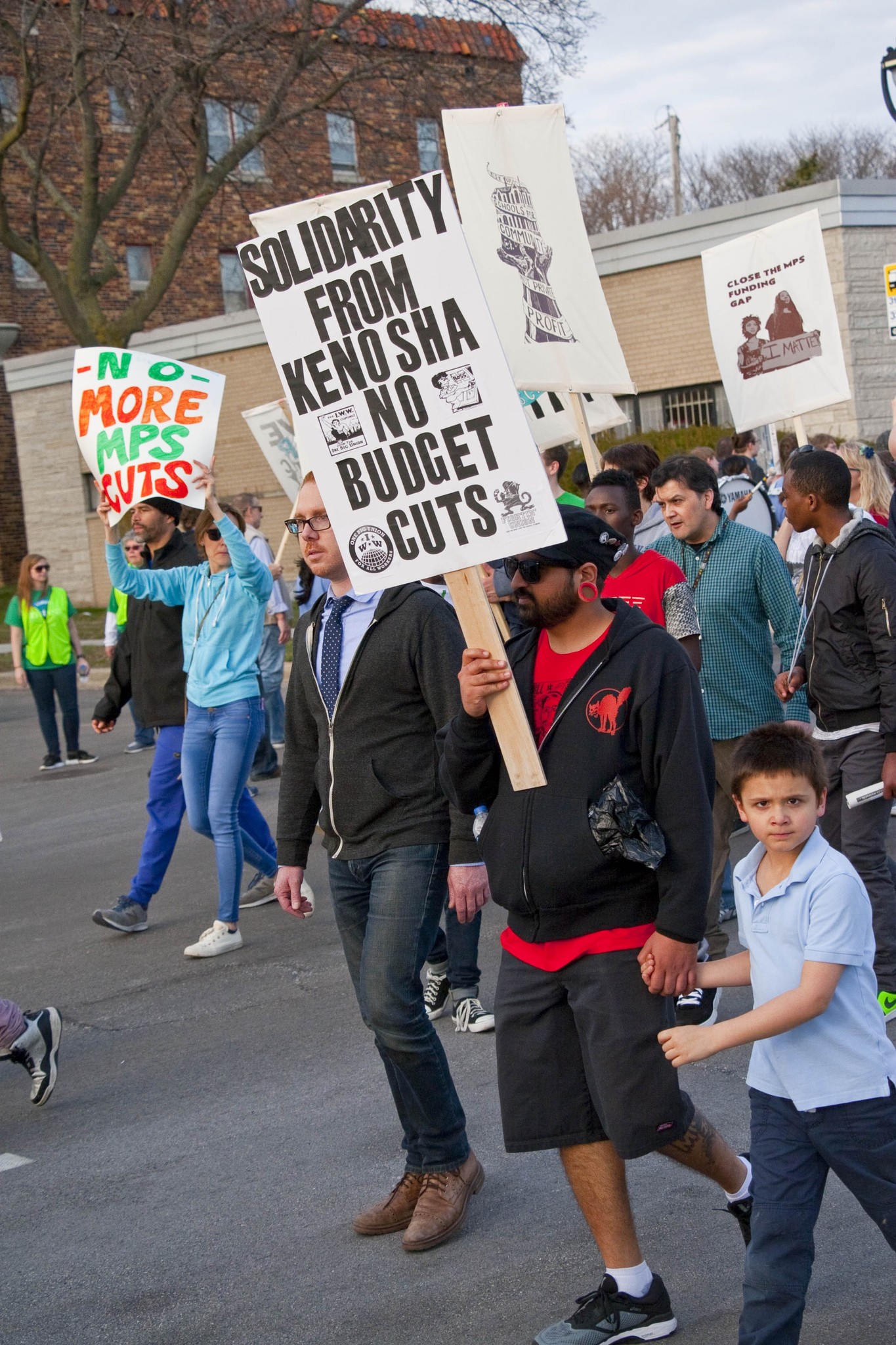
13 665 21 669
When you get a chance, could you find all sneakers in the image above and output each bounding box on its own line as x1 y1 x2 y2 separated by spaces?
184 919 244 957
532 1272 677 1345
238 872 278 909
38 753 65 770
301 877 315 917
452 997 495 1032
92 895 148 932
422 968 452 1021
125 739 156 753
674 954 722 1027
711 1152 754 1246
64 750 99 766
0 1006 62 1106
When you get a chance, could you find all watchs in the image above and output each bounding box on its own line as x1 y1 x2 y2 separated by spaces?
76 653 86 659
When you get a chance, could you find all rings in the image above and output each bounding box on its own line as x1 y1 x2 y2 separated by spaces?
204 469 212 476
99 507 102 512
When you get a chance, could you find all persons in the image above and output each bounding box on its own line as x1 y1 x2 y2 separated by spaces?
290 397 896 1034
4 554 99 771
273 472 491 1251
105 492 294 781
97 455 316 957
640 722 896 1345
92 498 289 935
459 505 754 1345
0 998 63 1106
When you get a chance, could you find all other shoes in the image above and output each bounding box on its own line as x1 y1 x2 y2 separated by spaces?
877 990 896 1023
252 766 281 781
719 907 738 921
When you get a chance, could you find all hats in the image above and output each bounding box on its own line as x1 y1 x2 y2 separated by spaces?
540 504 627 579
141 497 181 527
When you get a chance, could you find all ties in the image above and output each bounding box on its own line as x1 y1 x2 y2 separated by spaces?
318 596 358 723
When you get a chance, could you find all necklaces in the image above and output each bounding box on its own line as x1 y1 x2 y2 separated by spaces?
681 517 730 592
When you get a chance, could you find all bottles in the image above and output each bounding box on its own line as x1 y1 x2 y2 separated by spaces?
472 805 490 841
78 664 88 684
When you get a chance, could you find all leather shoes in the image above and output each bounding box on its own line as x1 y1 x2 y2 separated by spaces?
351 1148 486 1250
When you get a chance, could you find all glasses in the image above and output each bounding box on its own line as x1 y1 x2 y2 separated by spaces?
203 528 221 540
243 505 262 512
285 515 330 534
794 444 819 452
31 565 50 573
501 557 575 583
125 545 144 551
